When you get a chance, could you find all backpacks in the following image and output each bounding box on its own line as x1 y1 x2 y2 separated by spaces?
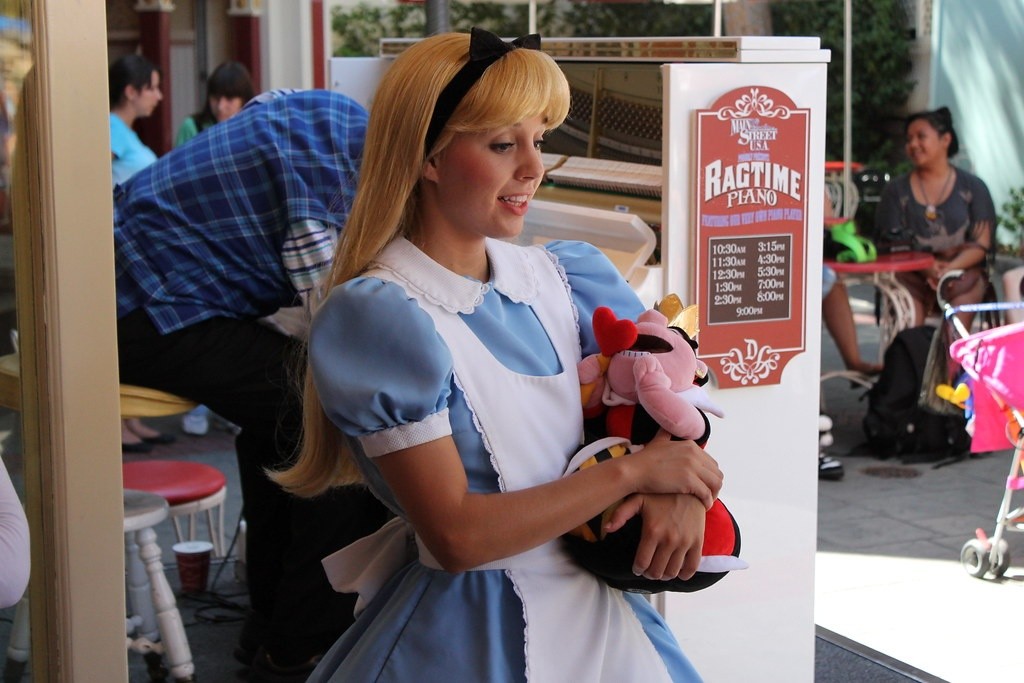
862 328 972 461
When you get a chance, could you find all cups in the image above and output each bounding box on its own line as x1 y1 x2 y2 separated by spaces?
172 541 214 593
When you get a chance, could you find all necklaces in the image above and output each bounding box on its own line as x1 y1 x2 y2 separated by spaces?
916 164 952 219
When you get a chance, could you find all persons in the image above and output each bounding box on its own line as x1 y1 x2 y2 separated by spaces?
0 456 31 609
268 31 726 683
875 106 998 402
176 62 255 435
113 92 369 667
824 186 883 387
109 59 178 452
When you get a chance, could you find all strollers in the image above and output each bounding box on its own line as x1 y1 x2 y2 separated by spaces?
936 270 1024 579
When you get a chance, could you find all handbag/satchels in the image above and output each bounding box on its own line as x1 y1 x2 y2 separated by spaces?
919 308 967 421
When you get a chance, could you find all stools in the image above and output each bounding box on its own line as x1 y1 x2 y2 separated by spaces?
7 488 194 683
126 459 229 567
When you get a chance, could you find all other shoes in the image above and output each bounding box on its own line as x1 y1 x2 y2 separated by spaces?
122 439 153 454
132 423 176 443
818 453 845 480
233 604 356 682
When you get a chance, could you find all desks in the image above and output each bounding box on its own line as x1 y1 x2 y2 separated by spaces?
828 254 935 366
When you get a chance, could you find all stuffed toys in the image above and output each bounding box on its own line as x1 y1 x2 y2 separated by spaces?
565 306 749 594
